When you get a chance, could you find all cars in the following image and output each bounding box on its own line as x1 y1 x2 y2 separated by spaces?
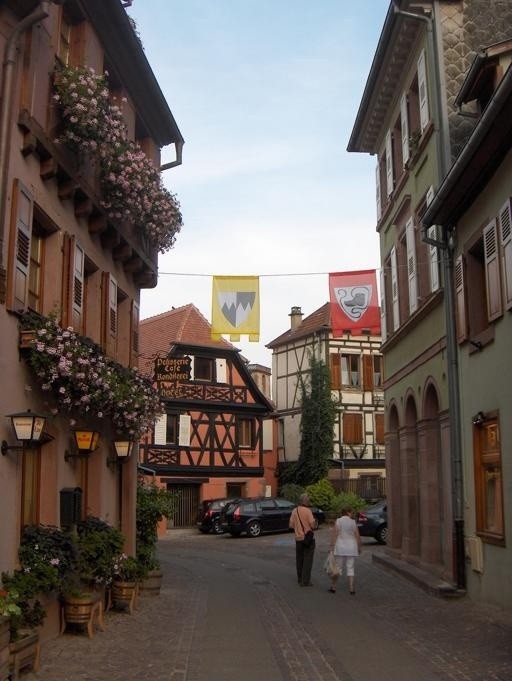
224 496 325 537
357 500 388 543
194 496 248 534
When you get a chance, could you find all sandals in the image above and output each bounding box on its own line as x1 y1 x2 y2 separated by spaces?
328 587 336 593
349 591 356 595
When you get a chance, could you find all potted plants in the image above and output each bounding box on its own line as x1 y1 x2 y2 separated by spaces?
58 553 165 640
8 598 49 681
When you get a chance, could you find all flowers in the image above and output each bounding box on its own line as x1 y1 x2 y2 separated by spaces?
20 310 166 441
51 59 188 255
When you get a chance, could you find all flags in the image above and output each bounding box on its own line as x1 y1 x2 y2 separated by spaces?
328 269 381 339
211 275 261 343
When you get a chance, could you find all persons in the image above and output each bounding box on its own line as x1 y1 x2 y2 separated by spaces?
329 508 361 595
289 493 318 587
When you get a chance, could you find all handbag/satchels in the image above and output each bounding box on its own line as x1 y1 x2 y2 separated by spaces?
303 531 314 541
324 551 340 577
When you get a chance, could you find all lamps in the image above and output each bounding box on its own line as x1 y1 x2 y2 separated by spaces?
64 425 135 468
0 409 50 458
